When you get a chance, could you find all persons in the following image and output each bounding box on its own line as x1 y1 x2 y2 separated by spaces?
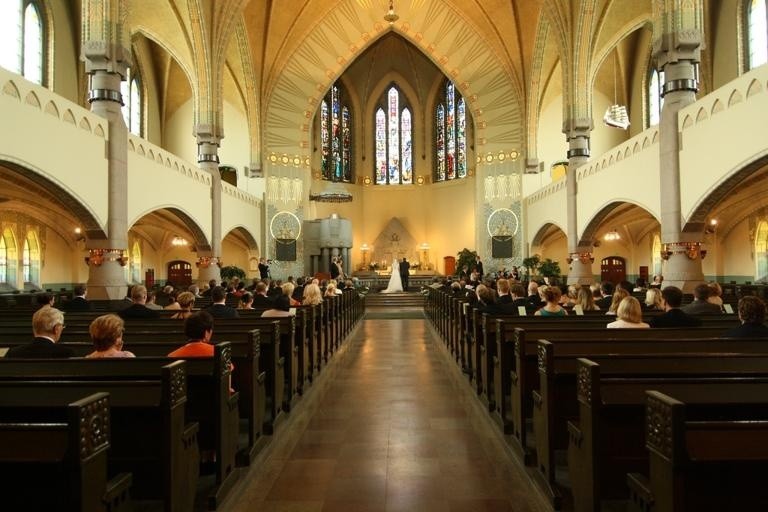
168 310 235 397
115 277 364 318
427 256 723 329
7 307 75 356
64 286 90 308
32 293 55 314
399 258 410 290
737 296 767 338
259 258 270 279
380 259 403 293
328 253 343 278
81 314 136 356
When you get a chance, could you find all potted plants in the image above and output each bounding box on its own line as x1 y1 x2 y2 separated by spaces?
523 254 539 276
221 265 246 282
538 259 560 286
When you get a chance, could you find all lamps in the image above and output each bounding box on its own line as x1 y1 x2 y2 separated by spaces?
602 48 631 131
171 235 187 246
603 227 620 242
383 0 399 26
